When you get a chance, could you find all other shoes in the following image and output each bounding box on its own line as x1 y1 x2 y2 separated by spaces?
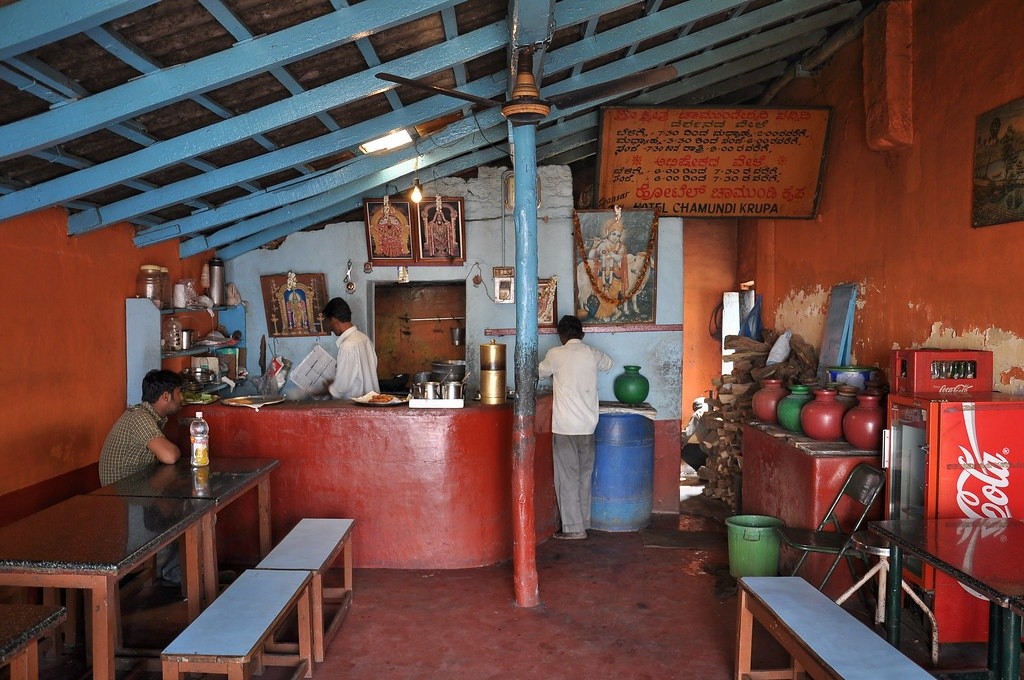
160 577 181 586
553 529 587 539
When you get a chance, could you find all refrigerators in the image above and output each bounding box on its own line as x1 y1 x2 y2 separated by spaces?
882 392 1023 643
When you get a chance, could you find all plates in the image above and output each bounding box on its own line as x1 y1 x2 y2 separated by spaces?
180 394 409 406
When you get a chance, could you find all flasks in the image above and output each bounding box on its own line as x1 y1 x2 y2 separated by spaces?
209 257 225 307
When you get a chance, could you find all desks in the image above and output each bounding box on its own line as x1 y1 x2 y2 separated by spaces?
0 496 217 680
741 417 889 603
0 603 68 680
86 455 282 607
869 519 1024 680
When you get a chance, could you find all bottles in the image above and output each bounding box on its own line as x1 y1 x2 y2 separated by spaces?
189 411 210 467
931 360 975 380
752 377 888 450
136 264 210 310
613 365 650 404
162 315 182 351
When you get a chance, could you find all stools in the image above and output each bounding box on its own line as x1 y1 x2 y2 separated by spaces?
836 531 940 667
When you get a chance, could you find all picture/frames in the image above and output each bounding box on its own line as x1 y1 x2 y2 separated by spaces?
363 197 467 266
571 207 659 326
536 279 559 328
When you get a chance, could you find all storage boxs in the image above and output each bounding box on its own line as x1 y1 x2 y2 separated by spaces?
889 348 993 394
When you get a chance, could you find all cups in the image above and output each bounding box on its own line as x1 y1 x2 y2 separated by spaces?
182 328 200 349
410 381 466 399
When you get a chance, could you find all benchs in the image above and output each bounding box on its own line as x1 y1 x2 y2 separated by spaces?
160 519 356 680
734 575 939 680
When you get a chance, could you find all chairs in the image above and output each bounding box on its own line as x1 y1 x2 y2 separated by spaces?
777 462 889 633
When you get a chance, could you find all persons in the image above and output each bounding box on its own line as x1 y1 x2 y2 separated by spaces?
681 397 718 482
321 297 380 400
113 465 191 531
539 316 614 539
98 369 236 586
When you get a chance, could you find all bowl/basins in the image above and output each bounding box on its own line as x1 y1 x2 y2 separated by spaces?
181 367 216 382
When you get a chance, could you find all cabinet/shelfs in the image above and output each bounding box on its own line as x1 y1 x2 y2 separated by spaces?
125 299 246 408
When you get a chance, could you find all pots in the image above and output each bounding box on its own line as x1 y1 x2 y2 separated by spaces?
379 373 408 391
412 359 465 383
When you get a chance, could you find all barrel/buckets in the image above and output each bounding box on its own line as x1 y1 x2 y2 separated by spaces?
591 413 655 532
725 514 784 578
450 320 465 345
825 365 880 391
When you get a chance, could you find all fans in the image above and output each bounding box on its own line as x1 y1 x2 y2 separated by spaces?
375 17 678 124
